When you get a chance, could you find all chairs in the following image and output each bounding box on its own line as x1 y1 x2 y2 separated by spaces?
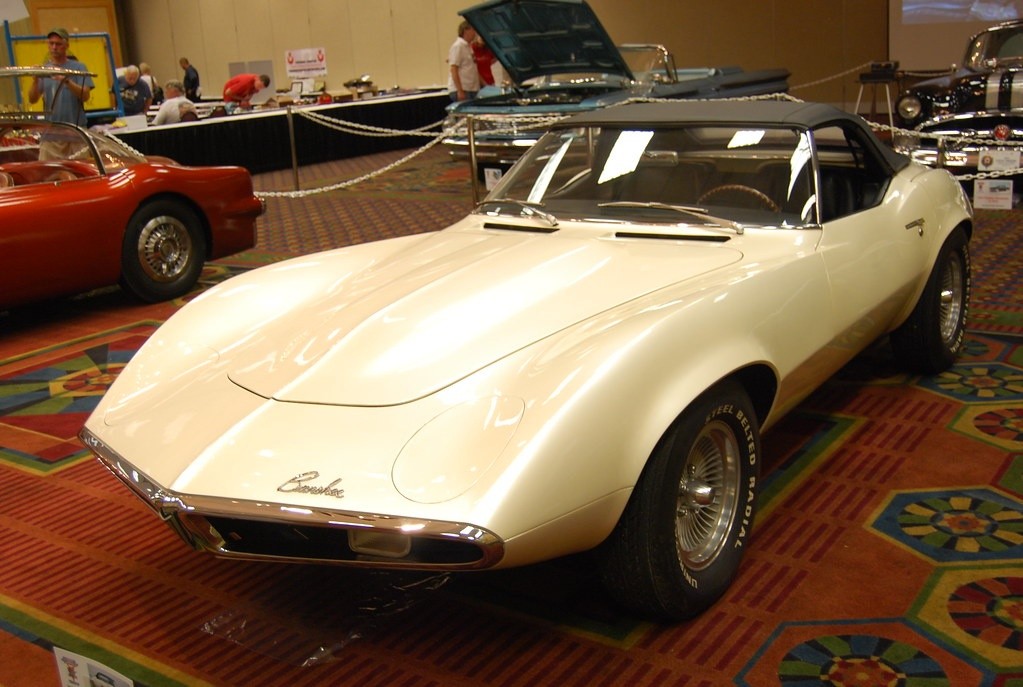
703 168 781 212
180 105 229 122
627 163 698 204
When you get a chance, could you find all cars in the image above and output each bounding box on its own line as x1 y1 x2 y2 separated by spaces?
895 21 1023 175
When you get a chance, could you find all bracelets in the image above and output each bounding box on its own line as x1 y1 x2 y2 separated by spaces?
113 106 116 110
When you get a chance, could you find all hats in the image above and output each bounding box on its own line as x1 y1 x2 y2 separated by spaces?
47 28 69 42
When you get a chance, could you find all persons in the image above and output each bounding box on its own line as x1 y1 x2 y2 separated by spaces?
28 29 96 163
112 64 152 118
138 62 161 105
180 57 202 103
151 80 198 127
446 20 499 104
223 71 271 115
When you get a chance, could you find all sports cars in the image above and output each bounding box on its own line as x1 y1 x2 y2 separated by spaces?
444 0 792 183
0 116 269 305
80 102 971 614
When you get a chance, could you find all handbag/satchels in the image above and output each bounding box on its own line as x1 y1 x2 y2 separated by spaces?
151 77 164 102
